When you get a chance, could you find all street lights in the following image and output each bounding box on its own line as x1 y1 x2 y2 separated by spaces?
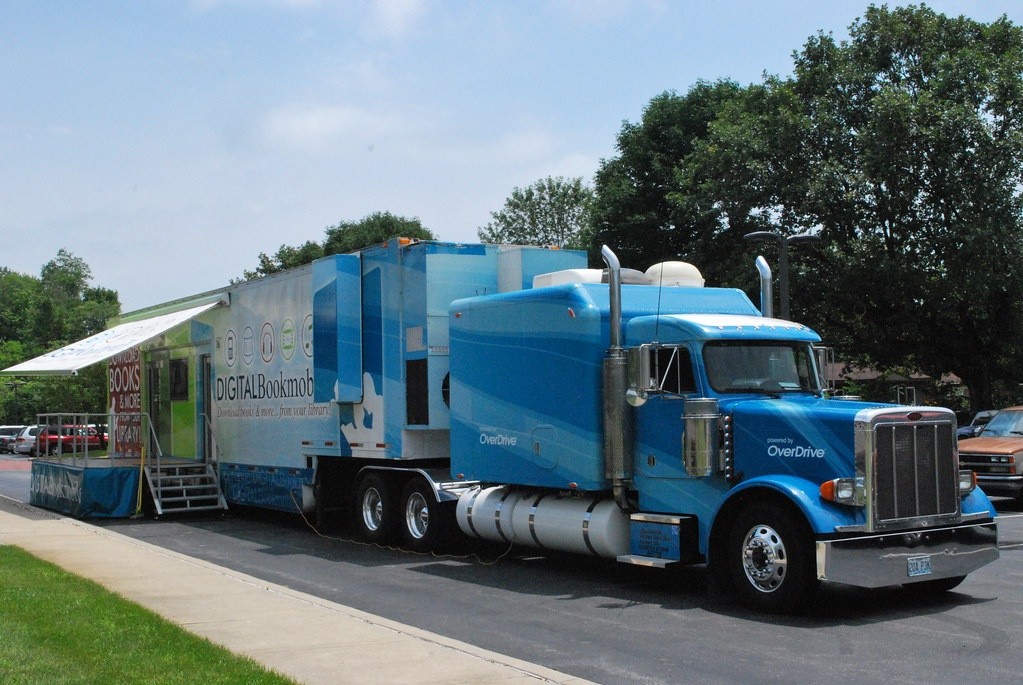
5 383 27 423
744 232 823 320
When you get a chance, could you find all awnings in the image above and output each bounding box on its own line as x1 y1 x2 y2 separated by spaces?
0 300 225 375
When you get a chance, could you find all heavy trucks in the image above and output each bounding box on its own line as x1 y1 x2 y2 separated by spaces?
0 235 1002 613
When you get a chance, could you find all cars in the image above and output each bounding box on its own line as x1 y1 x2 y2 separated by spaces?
958 410 1000 441
0 432 21 454
957 406 1022 513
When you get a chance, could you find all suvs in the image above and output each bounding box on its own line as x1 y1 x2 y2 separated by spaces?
14 424 50 455
34 423 109 456
0 425 28 454
